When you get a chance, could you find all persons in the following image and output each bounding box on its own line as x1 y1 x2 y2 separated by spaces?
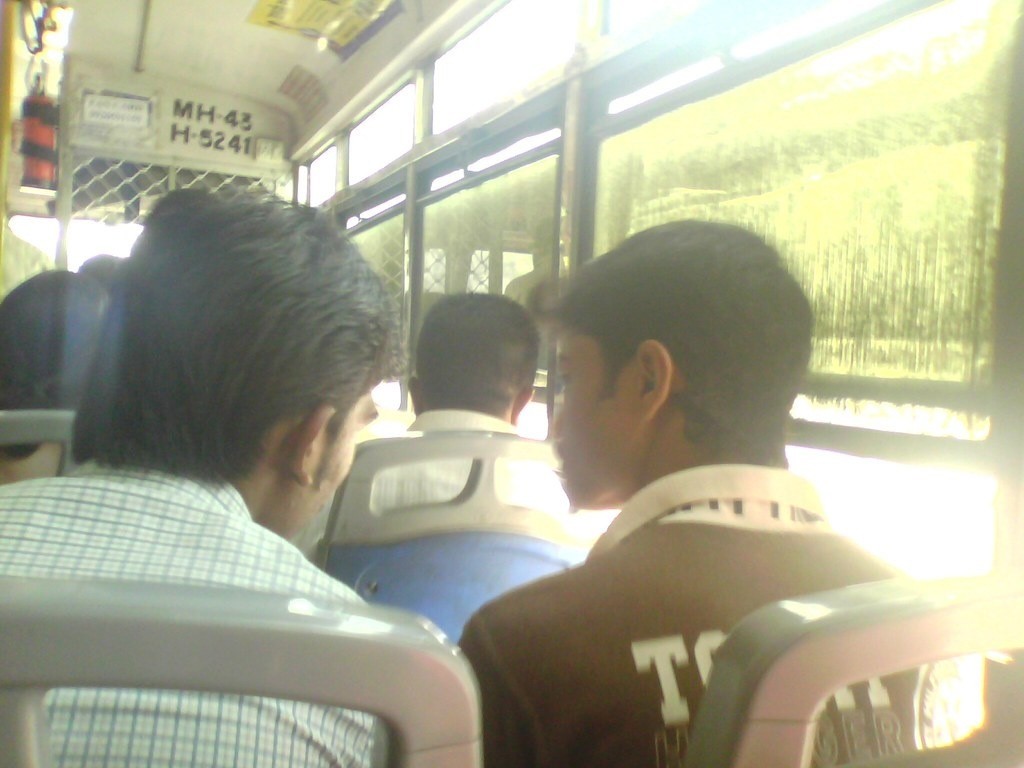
454 221 976 768
0 184 584 768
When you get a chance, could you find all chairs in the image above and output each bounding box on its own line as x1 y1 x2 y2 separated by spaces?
0 409 1024 768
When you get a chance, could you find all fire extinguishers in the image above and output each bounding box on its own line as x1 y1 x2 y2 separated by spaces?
21 74 57 189
